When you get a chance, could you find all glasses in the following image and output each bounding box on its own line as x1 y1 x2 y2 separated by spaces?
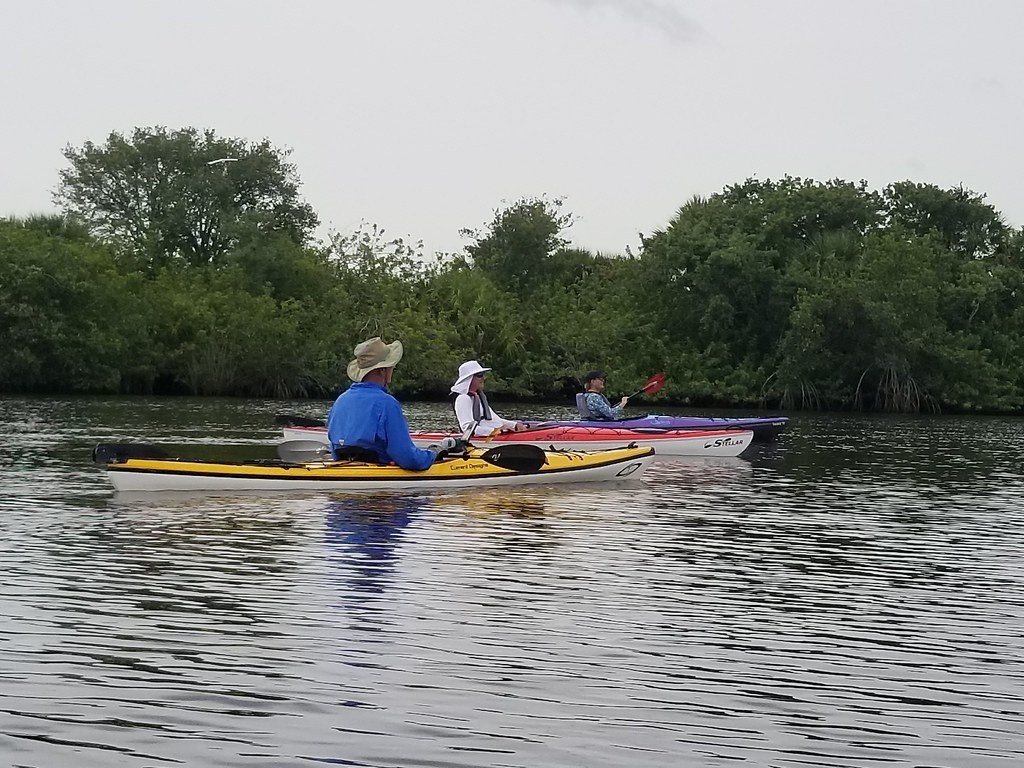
473 372 485 378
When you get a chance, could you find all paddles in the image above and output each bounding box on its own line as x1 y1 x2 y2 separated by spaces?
610 372 666 408
443 443 547 474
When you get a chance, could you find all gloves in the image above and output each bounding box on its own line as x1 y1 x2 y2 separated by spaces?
426 443 449 464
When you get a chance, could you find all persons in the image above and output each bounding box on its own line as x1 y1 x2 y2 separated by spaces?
450 360 528 436
327 337 436 472
584 372 628 422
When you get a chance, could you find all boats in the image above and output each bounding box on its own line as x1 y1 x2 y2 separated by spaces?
97 438 657 495
282 417 757 461
507 411 793 446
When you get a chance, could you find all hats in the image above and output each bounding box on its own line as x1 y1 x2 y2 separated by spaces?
454 361 493 386
346 335 403 383
586 370 609 381
